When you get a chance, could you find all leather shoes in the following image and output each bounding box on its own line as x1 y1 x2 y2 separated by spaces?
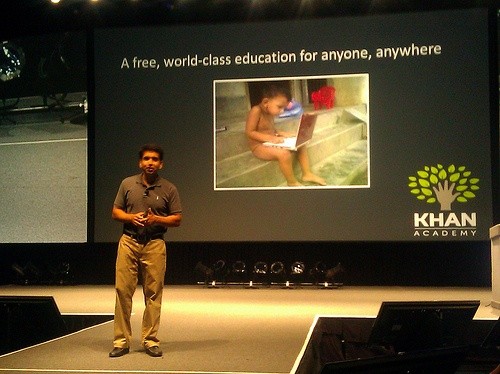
147 348 163 356
109 347 129 357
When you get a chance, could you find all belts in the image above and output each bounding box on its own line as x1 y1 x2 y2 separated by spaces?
122 231 162 241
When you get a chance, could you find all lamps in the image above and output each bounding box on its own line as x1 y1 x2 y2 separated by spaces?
193 259 345 288
11 260 78 286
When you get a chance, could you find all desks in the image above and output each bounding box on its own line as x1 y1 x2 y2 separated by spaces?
489 224 500 309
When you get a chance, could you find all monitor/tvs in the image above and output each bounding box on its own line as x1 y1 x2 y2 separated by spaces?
319 346 466 374
0 295 69 331
366 301 480 348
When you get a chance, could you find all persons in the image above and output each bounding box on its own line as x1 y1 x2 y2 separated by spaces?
245 84 327 186
108 145 182 358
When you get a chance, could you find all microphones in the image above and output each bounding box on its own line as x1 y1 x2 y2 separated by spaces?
145 190 149 196
